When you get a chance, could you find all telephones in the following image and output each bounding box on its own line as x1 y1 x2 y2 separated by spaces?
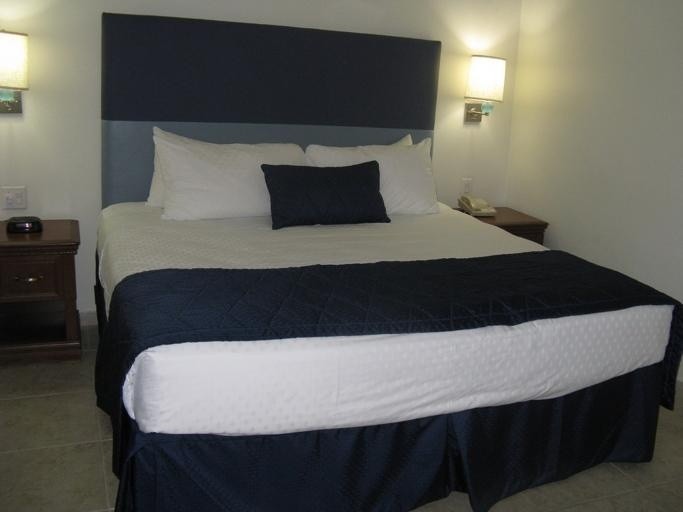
458 194 496 216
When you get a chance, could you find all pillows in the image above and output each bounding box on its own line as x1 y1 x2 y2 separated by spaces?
148 126 439 230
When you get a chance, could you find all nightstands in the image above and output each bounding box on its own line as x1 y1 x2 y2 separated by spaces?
0 218 84 364
450 206 550 245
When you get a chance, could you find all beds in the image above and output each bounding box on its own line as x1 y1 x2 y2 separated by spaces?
98 10 683 512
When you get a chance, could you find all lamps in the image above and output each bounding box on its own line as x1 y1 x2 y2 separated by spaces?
464 54 506 122
0 28 30 114
